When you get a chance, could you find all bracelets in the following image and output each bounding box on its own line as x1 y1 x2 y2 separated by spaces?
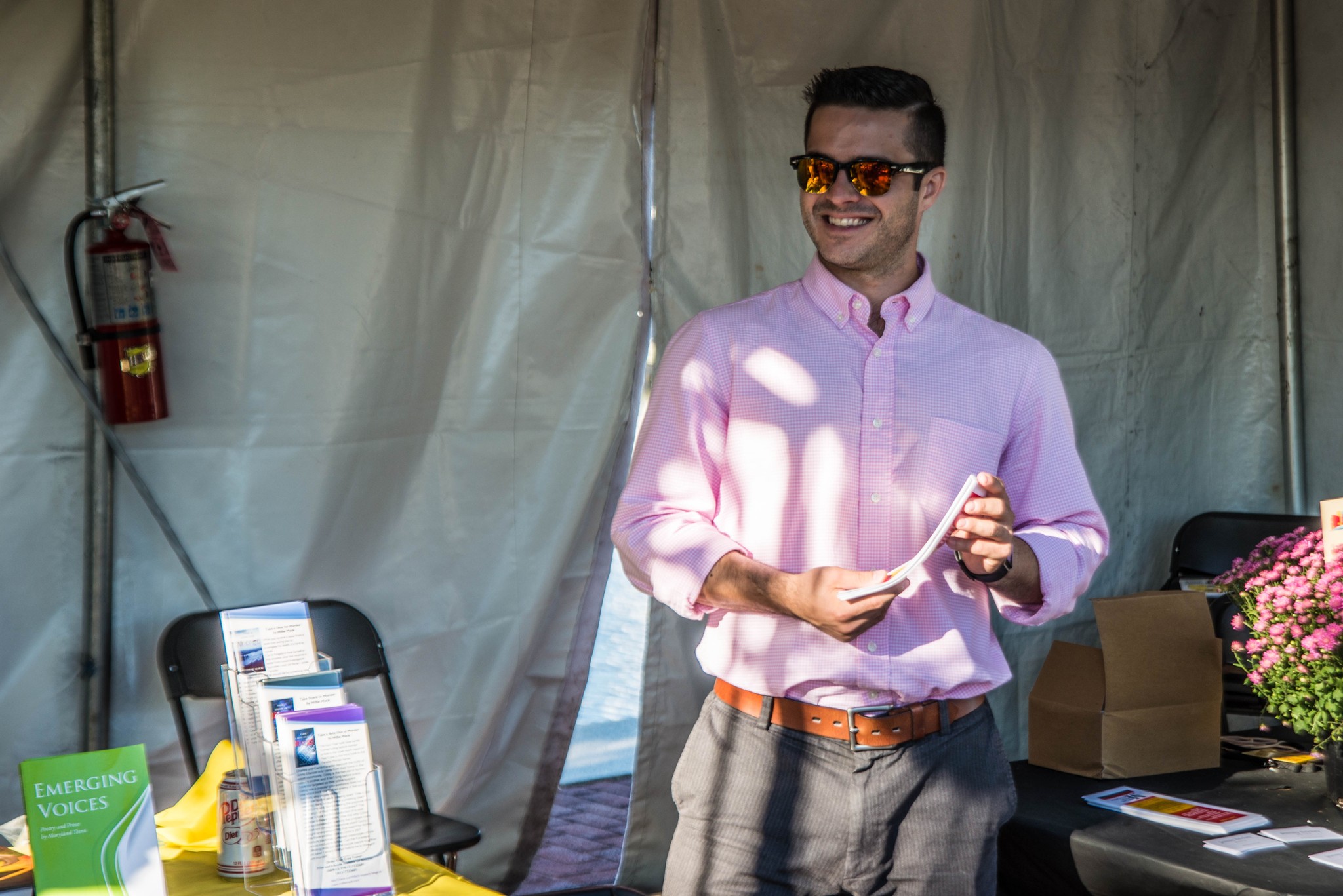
954 548 1013 583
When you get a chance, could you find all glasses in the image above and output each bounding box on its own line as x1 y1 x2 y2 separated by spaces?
790 154 933 197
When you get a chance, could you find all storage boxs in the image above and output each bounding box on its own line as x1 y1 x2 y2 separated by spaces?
1027 591 1225 780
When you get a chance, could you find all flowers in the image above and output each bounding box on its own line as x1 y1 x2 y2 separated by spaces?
1204 524 1343 756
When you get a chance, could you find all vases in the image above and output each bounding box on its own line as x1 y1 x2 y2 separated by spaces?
1323 728 1343 809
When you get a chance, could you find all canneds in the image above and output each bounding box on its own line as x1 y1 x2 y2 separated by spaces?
217 768 276 883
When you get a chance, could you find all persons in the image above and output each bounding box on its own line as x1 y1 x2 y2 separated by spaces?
610 66 1111 896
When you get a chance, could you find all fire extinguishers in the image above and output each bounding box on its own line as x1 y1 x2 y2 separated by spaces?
63 179 179 424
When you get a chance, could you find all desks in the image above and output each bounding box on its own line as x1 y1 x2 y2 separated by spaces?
997 738 1343 896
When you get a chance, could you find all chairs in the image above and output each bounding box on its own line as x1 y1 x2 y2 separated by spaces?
156 601 483 874
1160 513 1325 745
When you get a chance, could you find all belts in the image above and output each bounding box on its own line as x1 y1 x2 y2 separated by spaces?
713 677 985 751
